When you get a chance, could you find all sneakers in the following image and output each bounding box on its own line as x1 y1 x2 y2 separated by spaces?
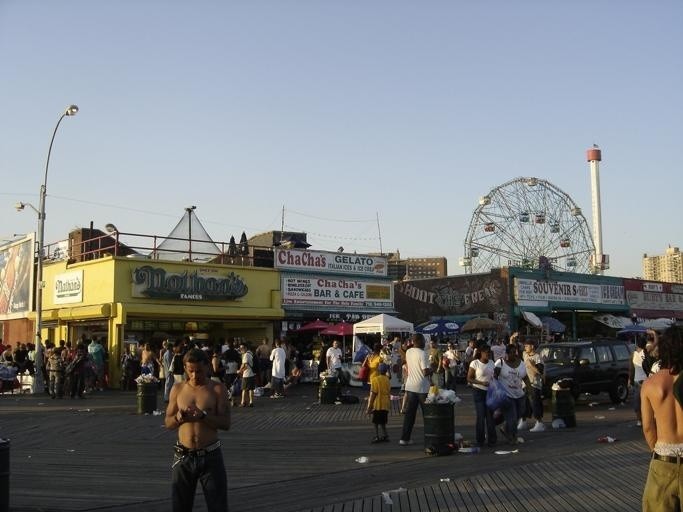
517 418 545 433
270 392 284 398
636 418 642 426
476 442 497 447
399 440 414 446
500 426 519 445
238 402 253 407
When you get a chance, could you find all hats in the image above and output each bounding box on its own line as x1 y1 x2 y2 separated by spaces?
378 363 388 373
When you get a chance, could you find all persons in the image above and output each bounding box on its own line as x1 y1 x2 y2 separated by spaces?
398 333 437 445
360 332 560 401
365 363 391 442
0 332 107 399
466 344 502 447
630 325 683 512
123 335 301 409
317 338 351 395
164 347 232 512
493 344 532 443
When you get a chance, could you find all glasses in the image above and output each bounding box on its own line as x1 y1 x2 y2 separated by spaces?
483 350 492 353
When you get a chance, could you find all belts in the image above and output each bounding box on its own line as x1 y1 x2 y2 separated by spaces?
184 439 222 458
651 451 683 463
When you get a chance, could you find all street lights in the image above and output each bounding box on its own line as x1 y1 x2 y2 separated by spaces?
12 104 77 393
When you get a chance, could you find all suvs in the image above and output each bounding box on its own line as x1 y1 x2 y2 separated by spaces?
526 339 636 402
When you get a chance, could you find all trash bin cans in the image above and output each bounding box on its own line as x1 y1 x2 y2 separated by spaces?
136 383 159 415
319 376 339 404
551 390 576 429
0 437 11 512
420 401 456 456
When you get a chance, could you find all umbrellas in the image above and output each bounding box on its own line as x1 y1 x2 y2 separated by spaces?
638 318 673 331
541 316 566 333
615 322 649 345
413 318 463 336
593 314 625 329
319 318 355 363
521 310 543 330
461 316 497 332
299 317 332 335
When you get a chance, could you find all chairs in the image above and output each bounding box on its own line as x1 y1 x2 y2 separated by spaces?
580 348 594 364
558 352 568 361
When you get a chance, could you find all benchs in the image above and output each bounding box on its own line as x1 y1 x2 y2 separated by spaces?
591 346 624 361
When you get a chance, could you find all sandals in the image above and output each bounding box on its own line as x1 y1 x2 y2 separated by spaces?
371 435 389 443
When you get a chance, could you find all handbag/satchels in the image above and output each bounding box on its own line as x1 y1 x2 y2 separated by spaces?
642 357 657 377
359 363 369 382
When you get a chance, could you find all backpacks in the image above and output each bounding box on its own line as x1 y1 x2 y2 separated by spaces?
246 351 259 373
